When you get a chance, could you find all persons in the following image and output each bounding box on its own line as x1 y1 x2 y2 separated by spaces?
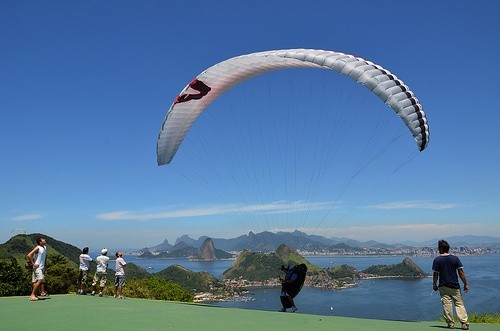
76 247 99 296
285 263 308 312
90 248 110 297
113 251 128 299
432 240 470 330
279 264 298 312
26 236 50 301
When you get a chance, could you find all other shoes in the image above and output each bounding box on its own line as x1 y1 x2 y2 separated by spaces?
78 289 81 293
99 293 103 296
462 323 469 329
279 308 286 311
120 296 123 299
29 295 39 300
290 307 297 312
80 291 87 295
114 294 117 298
41 292 47 295
447 325 454 328
90 292 95 296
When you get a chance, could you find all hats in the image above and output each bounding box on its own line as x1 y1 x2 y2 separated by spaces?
101 248 108 254
